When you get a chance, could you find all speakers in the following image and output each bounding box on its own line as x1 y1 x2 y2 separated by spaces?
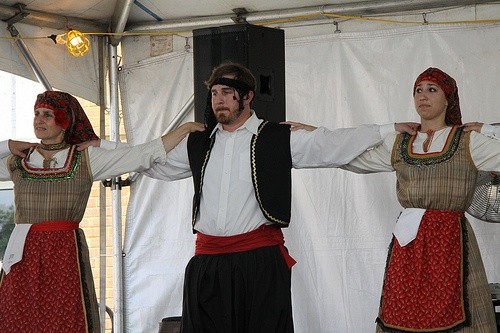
192 23 285 125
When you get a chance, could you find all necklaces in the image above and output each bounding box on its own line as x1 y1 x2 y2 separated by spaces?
40 139 67 150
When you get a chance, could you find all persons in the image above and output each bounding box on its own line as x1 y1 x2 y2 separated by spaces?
0 139 40 160
76 63 423 333
0 90 209 333
460 121 500 141
277 68 500 333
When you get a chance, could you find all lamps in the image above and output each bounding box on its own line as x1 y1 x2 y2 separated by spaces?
66 26 90 57
